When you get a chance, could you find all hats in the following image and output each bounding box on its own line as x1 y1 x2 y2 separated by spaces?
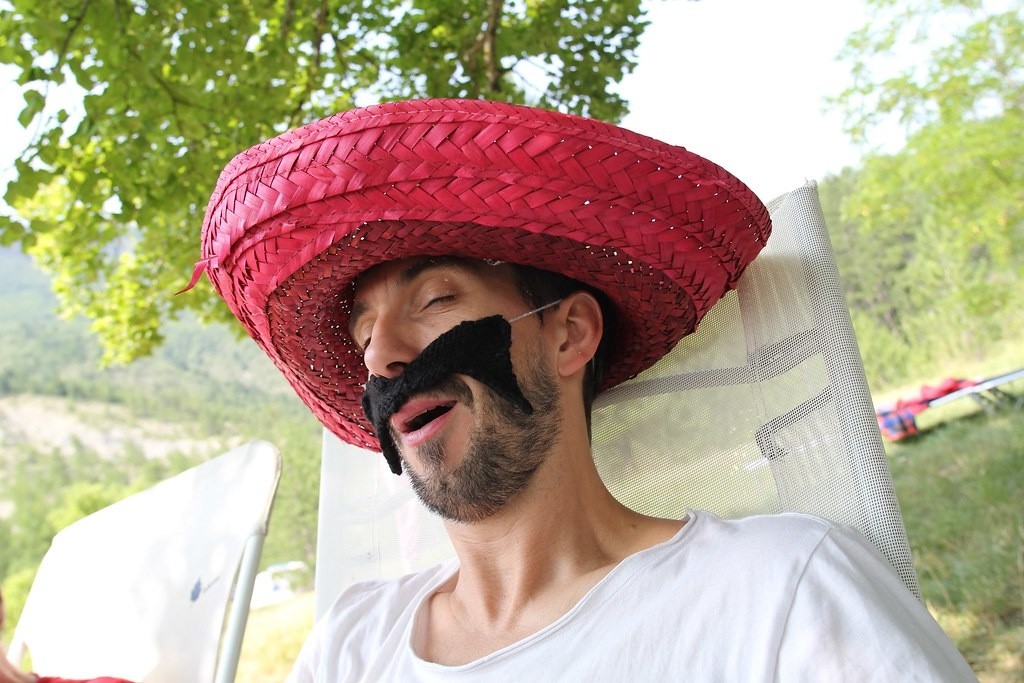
175 93 773 453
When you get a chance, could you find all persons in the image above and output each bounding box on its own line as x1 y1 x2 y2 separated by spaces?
172 100 979 683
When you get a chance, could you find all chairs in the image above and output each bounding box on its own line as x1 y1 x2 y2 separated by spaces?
6 439 284 683
310 180 921 616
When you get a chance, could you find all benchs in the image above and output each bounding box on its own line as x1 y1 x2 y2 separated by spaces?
749 368 1024 475
